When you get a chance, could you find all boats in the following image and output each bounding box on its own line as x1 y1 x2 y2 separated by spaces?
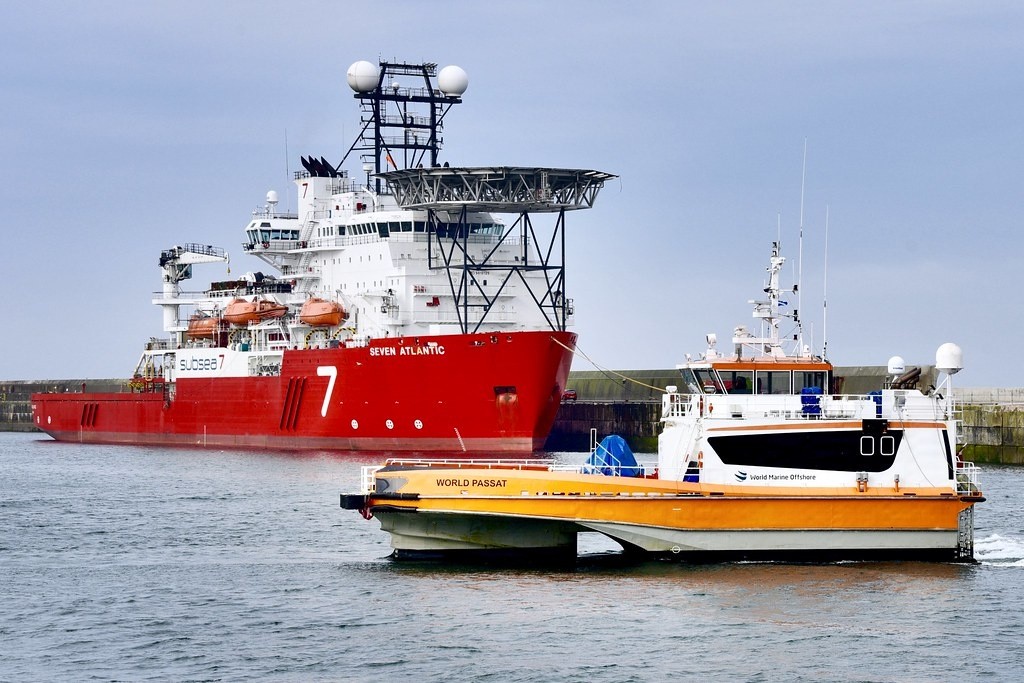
223 297 261 324
253 300 289 319
297 297 349 327
338 139 987 566
28 51 621 461
186 313 223 340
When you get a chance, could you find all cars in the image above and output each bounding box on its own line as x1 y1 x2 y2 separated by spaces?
560 389 580 402
